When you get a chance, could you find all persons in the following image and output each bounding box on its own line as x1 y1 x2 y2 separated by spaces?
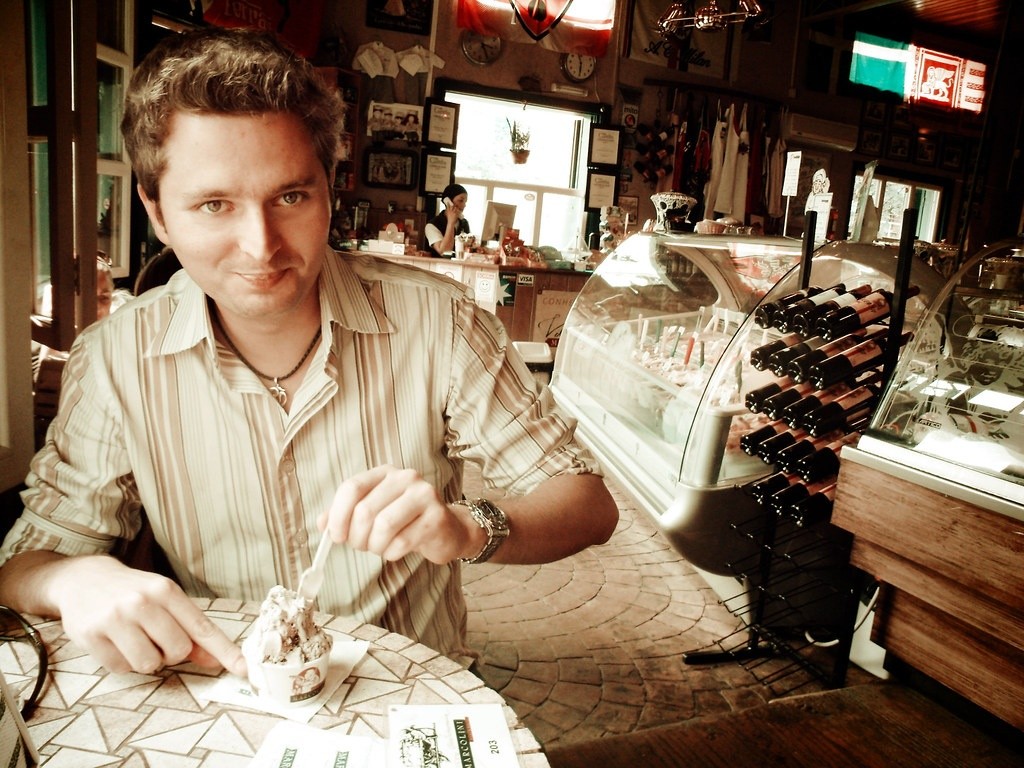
423 182 471 259
38 253 114 360
0 30 620 693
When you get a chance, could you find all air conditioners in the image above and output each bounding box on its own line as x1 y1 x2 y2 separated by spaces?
785 112 859 153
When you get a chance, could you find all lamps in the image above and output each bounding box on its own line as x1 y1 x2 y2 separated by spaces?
649 0 769 41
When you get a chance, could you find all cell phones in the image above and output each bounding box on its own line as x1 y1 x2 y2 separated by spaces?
443 196 455 206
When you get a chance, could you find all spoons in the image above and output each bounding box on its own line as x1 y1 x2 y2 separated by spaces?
296 527 334 601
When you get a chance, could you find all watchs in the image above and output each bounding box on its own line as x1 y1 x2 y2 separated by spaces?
454 497 512 564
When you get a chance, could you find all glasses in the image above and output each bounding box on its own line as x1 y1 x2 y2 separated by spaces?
0 605 47 721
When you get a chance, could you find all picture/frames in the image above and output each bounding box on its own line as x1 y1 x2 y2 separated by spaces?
585 168 621 213
889 101 916 136
741 0 777 46
858 98 888 130
885 131 912 163
418 148 457 198
366 99 425 142
587 122 626 169
421 97 461 150
855 125 885 158
619 195 640 226
937 139 964 174
365 0 434 36
912 135 940 169
361 146 420 191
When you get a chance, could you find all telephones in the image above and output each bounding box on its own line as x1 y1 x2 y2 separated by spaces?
443 197 465 220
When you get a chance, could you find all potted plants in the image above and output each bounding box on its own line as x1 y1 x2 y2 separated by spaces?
505 116 531 164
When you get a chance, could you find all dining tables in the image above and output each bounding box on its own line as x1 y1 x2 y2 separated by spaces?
0 595 551 768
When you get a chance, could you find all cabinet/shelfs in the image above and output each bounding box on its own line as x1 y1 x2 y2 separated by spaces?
313 65 362 193
681 207 922 701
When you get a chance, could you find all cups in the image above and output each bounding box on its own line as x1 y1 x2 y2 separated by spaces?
405 219 414 232
455 234 474 261
355 206 368 230
241 637 333 706
995 274 1009 289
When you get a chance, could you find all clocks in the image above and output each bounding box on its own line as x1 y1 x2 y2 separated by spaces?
558 45 597 85
459 24 505 69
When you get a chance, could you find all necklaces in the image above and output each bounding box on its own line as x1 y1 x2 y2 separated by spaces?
211 309 321 408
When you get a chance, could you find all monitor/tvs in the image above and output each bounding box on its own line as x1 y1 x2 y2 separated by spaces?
480 199 515 253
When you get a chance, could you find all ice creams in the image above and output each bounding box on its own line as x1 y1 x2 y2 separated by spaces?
244 584 332 665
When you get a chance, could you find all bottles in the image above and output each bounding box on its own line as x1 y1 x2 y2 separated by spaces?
648 127 674 144
643 165 673 183
644 145 675 164
723 281 922 603
